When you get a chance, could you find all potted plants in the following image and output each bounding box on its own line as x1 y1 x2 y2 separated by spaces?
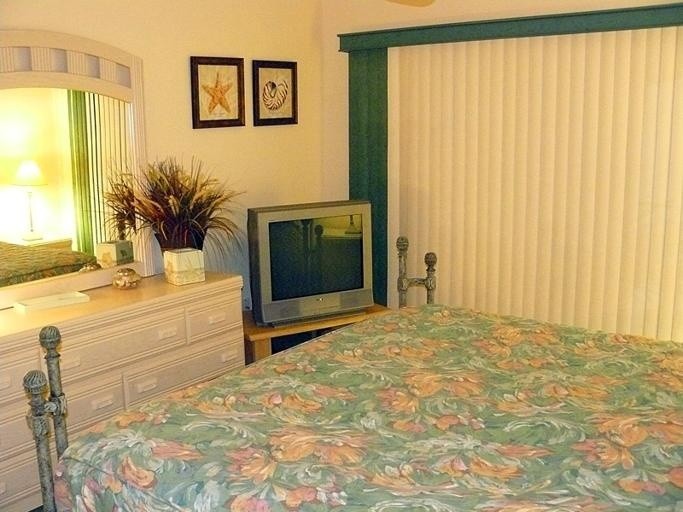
97 161 246 286
87 175 137 268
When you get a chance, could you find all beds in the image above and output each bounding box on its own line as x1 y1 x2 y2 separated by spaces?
0 241 97 288
24 238 682 512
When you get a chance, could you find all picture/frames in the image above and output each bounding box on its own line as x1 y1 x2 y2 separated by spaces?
192 56 299 127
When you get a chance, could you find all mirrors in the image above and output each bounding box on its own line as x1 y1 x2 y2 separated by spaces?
0 30 148 314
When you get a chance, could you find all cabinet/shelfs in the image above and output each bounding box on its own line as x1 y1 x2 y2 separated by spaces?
1 268 242 512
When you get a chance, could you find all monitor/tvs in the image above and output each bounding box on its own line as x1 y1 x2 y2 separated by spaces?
246 198 375 329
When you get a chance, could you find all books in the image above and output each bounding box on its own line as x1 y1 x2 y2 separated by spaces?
12 289 89 312
